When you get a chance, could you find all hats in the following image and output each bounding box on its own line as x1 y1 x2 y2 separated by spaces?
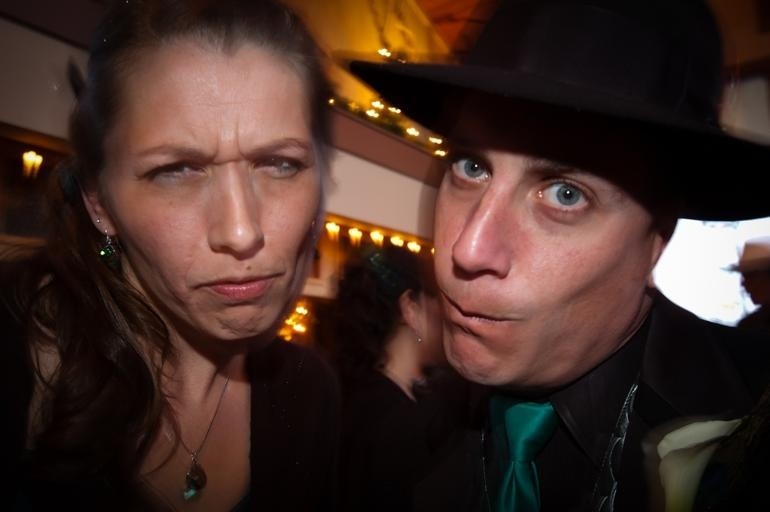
350 3 770 221
721 236 769 274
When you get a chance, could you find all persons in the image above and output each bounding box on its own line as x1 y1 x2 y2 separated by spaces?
332 0 769 512
315 237 444 512
2 1 351 512
726 239 769 339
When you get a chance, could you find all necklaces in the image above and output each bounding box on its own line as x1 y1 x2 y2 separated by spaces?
151 351 239 503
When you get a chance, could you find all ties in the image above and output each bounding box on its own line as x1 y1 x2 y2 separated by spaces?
495 402 560 510
